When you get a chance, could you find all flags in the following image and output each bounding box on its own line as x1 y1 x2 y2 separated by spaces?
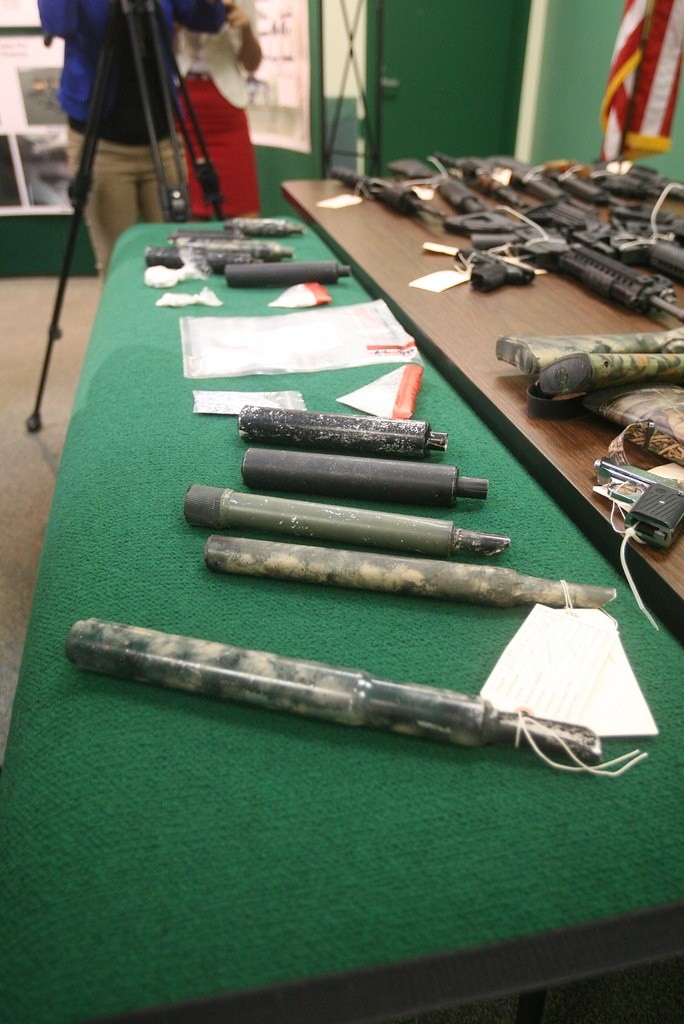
597 0 684 168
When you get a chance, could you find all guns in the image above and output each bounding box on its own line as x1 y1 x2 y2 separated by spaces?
328 151 683 333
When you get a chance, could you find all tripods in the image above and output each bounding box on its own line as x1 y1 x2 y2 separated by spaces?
24 0 222 435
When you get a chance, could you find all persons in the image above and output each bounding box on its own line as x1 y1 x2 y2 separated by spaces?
170 0 260 223
37 0 234 281
